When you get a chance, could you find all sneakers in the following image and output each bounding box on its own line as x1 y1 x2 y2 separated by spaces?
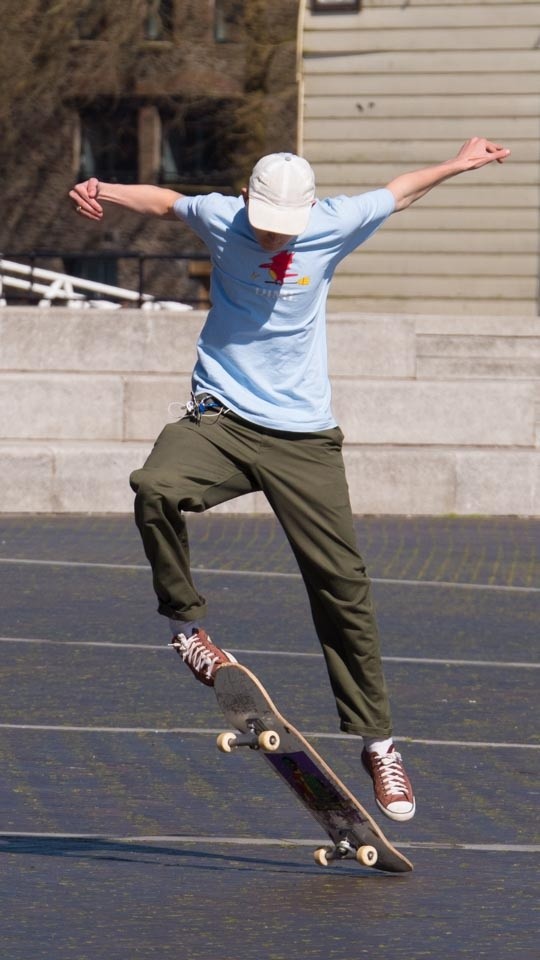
167 627 237 688
361 744 416 821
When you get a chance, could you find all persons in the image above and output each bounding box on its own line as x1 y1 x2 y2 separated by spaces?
65 136 513 824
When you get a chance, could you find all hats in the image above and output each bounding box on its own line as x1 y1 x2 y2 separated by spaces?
247 153 315 236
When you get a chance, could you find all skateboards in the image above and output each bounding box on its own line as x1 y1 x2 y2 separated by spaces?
212 662 414 873
